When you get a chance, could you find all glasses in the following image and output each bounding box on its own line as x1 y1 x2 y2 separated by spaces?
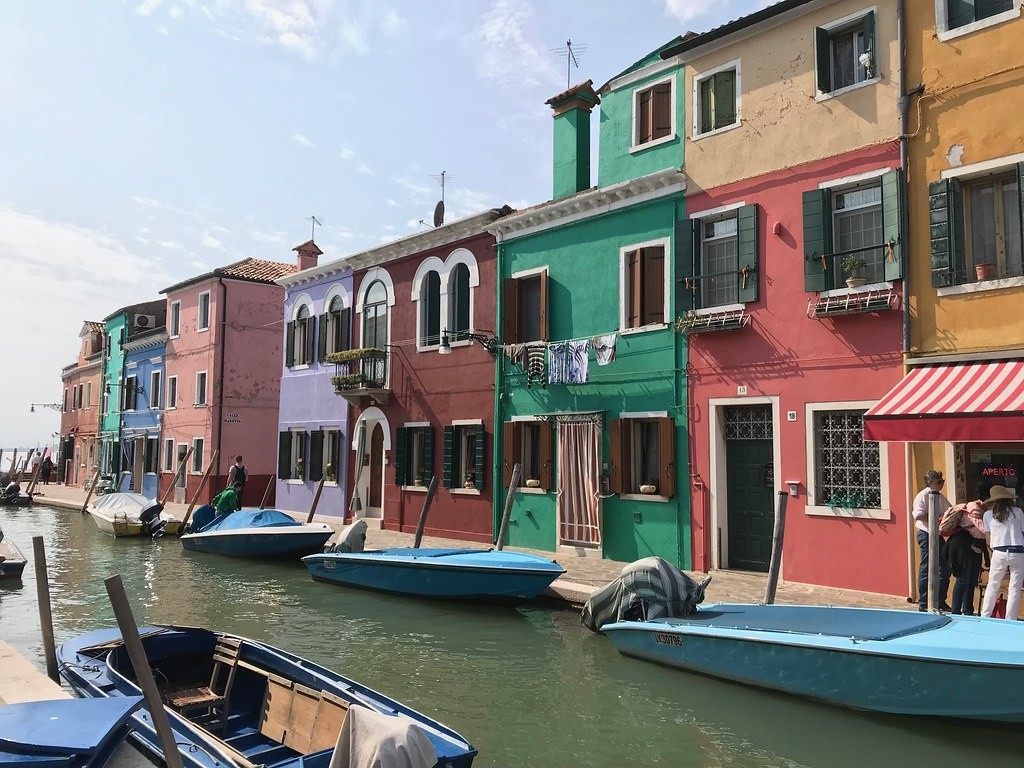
935 480 945 485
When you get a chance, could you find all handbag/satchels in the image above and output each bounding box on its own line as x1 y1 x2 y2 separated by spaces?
980 544 994 571
990 593 1007 619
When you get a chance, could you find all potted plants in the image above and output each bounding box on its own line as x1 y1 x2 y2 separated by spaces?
841 255 868 287
526 476 541 488
640 480 656 494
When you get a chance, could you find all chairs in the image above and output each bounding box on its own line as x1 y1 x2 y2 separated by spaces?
164 635 242 737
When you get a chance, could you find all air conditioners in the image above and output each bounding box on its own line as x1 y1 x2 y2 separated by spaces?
134 312 155 328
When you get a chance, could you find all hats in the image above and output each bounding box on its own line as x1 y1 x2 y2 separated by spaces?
982 485 1018 508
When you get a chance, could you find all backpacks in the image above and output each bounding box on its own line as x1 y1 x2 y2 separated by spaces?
232 465 246 485
936 502 984 536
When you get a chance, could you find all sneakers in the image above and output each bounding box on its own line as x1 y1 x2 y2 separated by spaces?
918 604 936 612
938 602 952 612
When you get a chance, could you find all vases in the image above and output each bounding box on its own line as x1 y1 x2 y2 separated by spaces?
326 474 336 481
299 474 304 480
975 263 996 280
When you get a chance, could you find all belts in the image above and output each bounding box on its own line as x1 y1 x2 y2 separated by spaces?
993 547 1024 553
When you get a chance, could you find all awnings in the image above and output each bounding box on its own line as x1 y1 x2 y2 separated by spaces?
862 357 1024 442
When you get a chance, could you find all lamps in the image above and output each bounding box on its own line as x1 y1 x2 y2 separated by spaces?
439 326 498 357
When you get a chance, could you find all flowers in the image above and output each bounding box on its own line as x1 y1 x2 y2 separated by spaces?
324 459 335 475
295 458 305 476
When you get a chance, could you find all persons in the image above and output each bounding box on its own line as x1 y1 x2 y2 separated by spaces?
911 469 953 612
942 469 1024 622
30 452 43 485
225 455 248 511
40 456 54 485
210 480 244 517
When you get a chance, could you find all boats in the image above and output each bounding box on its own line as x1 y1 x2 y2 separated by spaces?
581 556 1024 724
56 623 479 768
0 530 28 577
86 497 181 537
301 547 567 609
181 522 336 558
0 482 33 506
